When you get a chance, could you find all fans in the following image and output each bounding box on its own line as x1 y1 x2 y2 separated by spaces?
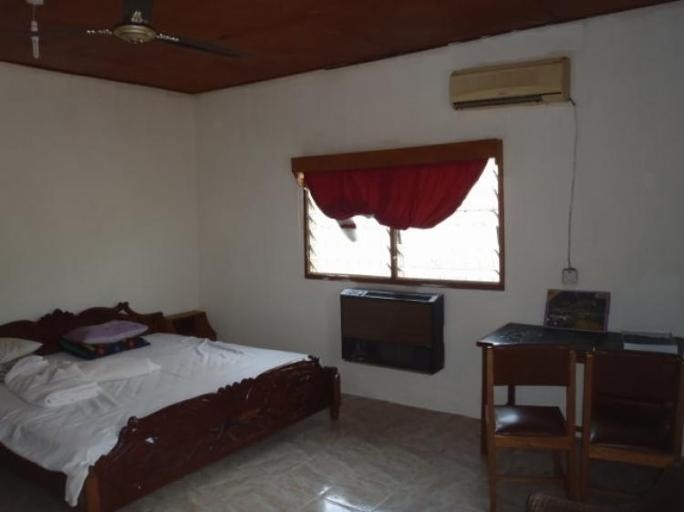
46 0 241 59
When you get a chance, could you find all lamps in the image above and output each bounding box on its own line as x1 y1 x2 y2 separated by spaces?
25 0 43 59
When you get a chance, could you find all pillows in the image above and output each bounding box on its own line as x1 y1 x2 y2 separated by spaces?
60 320 152 360
0 338 42 381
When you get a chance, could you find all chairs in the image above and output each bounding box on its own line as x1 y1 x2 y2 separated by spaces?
483 343 684 512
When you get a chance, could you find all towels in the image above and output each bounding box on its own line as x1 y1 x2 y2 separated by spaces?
0 302 342 512
72 348 160 382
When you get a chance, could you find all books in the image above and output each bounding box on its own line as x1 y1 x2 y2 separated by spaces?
623 333 677 355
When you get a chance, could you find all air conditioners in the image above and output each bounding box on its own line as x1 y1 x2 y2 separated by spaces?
449 57 569 111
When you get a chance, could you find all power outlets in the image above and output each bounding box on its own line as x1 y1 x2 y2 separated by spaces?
562 268 577 283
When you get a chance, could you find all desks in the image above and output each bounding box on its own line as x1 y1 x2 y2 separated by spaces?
475 321 683 459
